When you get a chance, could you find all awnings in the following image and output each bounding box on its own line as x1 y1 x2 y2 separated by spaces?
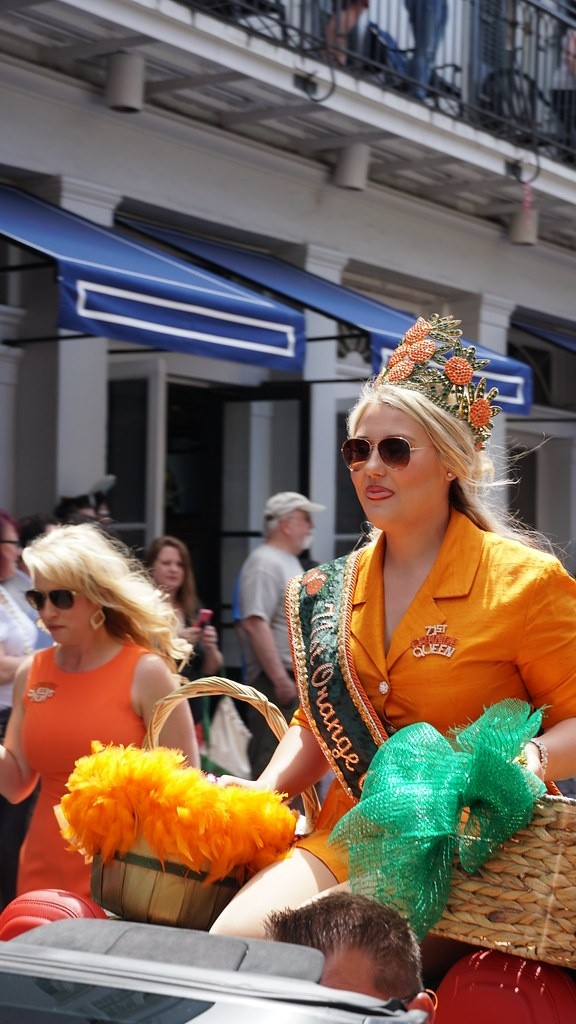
0 183 307 370
512 318 576 357
116 216 532 414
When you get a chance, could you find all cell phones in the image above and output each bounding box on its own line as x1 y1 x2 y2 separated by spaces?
192 609 214 629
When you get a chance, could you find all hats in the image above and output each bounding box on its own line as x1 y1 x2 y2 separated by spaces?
263 491 326 517
59 475 116 508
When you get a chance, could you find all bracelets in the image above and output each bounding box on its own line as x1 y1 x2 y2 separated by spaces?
530 738 548 777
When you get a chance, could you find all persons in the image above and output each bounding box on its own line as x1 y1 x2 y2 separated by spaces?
0 473 225 917
316 0 369 68
264 892 436 1024
1 525 201 921
228 493 329 783
405 0 448 106
205 314 575 940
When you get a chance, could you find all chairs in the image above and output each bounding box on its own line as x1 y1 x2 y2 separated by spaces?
180 0 570 160
424 946 576 1024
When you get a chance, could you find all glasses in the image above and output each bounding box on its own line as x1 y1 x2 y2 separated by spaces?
0 538 25 546
24 589 81 611
340 436 426 472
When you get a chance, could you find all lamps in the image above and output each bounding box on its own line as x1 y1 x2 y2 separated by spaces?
509 203 540 246
332 143 372 191
105 51 149 114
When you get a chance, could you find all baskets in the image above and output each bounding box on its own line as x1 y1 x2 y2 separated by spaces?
351 792 576 969
89 676 321 926
